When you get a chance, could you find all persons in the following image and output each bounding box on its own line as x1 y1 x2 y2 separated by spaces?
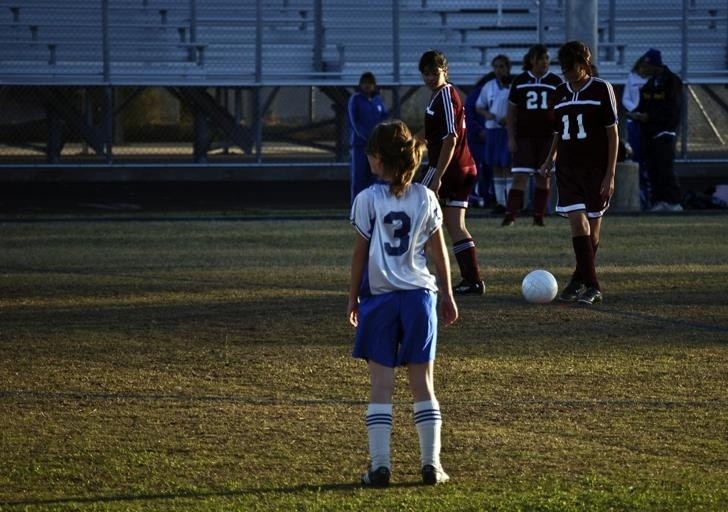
635 50 684 213
540 40 619 304
346 71 387 200
465 44 602 226
418 51 486 295
622 56 657 207
348 121 459 485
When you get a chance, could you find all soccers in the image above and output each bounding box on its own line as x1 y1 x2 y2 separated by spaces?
522 271 559 303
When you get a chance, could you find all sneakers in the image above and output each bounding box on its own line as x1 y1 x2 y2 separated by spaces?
559 278 584 301
490 205 545 226
422 465 450 485
578 291 602 305
361 465 390 488
651 202 683 212
453 281 485 295
